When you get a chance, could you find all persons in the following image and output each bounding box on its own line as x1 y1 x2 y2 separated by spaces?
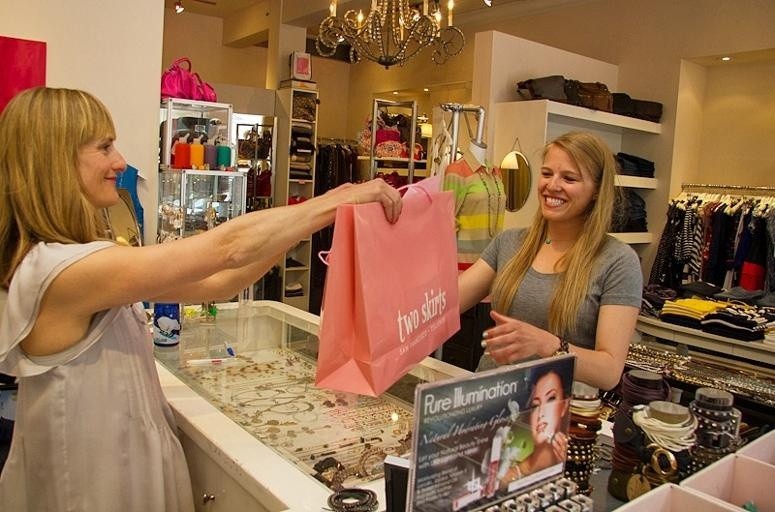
457 130 644 395
1 83 402 511
441 137 507 374
494 364 570 493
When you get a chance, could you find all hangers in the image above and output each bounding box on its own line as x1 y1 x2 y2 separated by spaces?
671 183 775 215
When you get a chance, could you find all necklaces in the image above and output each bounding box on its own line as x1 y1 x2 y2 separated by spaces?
476 168 502 237
188 341 414 492
625 340 775 416
543 225 580 244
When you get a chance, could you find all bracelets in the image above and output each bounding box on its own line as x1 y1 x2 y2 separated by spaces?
328 488 378 512
562 369 746 498
552 335 570 358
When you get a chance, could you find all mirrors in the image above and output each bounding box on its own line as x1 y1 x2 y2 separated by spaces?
500 150 532 212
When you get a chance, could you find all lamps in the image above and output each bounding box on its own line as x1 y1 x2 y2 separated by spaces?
314 0 467 70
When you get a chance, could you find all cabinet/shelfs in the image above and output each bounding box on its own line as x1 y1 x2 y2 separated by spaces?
157 87 317 312
492 100 662 245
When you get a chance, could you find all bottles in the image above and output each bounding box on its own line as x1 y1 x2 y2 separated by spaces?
152 303 182 347
173 133 232 170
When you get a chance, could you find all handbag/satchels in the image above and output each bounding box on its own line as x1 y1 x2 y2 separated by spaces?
239 139 270 159
161 57 217 102
315 174 461 399
518 75 662 123
